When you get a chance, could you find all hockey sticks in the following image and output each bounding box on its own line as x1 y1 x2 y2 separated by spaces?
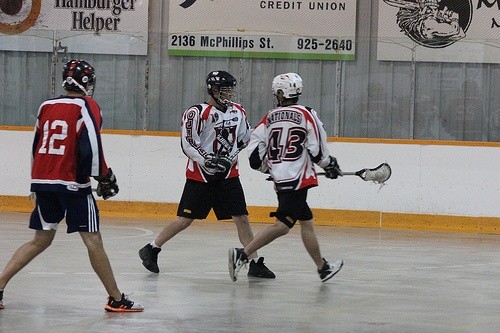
317 164 391 185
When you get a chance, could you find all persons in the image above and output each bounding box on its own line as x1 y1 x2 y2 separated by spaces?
227 71 344 284
138 70 278 279
353 73 490 145
0 58 146 313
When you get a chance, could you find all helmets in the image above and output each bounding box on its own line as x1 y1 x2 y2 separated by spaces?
61 59 95 98
206 70 238 109
271 72 303 99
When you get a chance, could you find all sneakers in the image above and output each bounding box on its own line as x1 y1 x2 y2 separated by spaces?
0 290 5 309
104 292 145 312
247 256 276 279
228 248 249 282
138 243 162 273
316 258 343 282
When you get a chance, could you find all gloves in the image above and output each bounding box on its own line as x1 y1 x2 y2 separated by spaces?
323 155 343 179
93 167 119 200
204 154 236 179
